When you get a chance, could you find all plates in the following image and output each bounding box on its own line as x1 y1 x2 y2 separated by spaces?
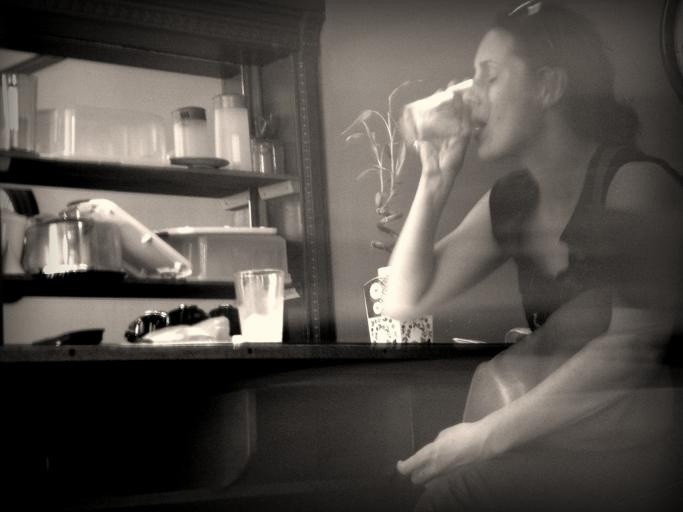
169 156 229 169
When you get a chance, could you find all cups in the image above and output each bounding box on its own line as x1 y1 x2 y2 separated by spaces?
402 78 490 141
0 72 39 154
233 268 285 343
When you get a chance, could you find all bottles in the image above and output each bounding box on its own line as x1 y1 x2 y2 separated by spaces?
172 106 208 159
215 93 276 177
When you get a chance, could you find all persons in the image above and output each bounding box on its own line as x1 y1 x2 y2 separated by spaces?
381 0 683 512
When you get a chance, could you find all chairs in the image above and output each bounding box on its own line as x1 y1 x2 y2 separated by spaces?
403 284 683 512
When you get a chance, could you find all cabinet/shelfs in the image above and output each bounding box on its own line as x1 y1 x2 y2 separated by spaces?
0 0 340 344
